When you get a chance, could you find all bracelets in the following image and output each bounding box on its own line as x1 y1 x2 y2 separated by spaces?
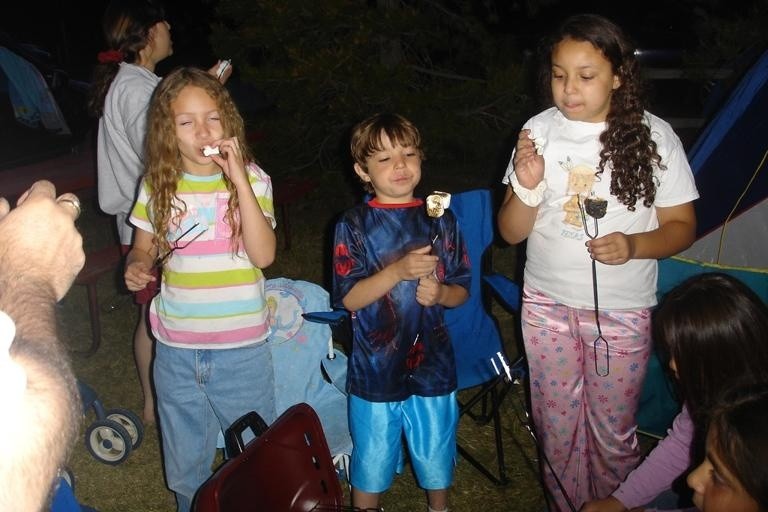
507 170 548 208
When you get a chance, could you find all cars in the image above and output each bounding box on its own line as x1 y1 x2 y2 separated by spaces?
629 45 736 147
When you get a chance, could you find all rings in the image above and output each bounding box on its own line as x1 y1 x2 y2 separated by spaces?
53 196 83 218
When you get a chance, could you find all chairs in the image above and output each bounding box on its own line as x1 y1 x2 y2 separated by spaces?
1 153 135 356
307 192 535 489
1 35 110 159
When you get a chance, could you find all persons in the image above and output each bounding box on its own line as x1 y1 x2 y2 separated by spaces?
2 178 88 512
495 15 702 510
119 66 279 511
332 113 472 510
90 2 232 427
682 371 768 510
580 270 768 511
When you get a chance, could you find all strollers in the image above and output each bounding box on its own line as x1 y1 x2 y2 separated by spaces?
56 370 144 500
